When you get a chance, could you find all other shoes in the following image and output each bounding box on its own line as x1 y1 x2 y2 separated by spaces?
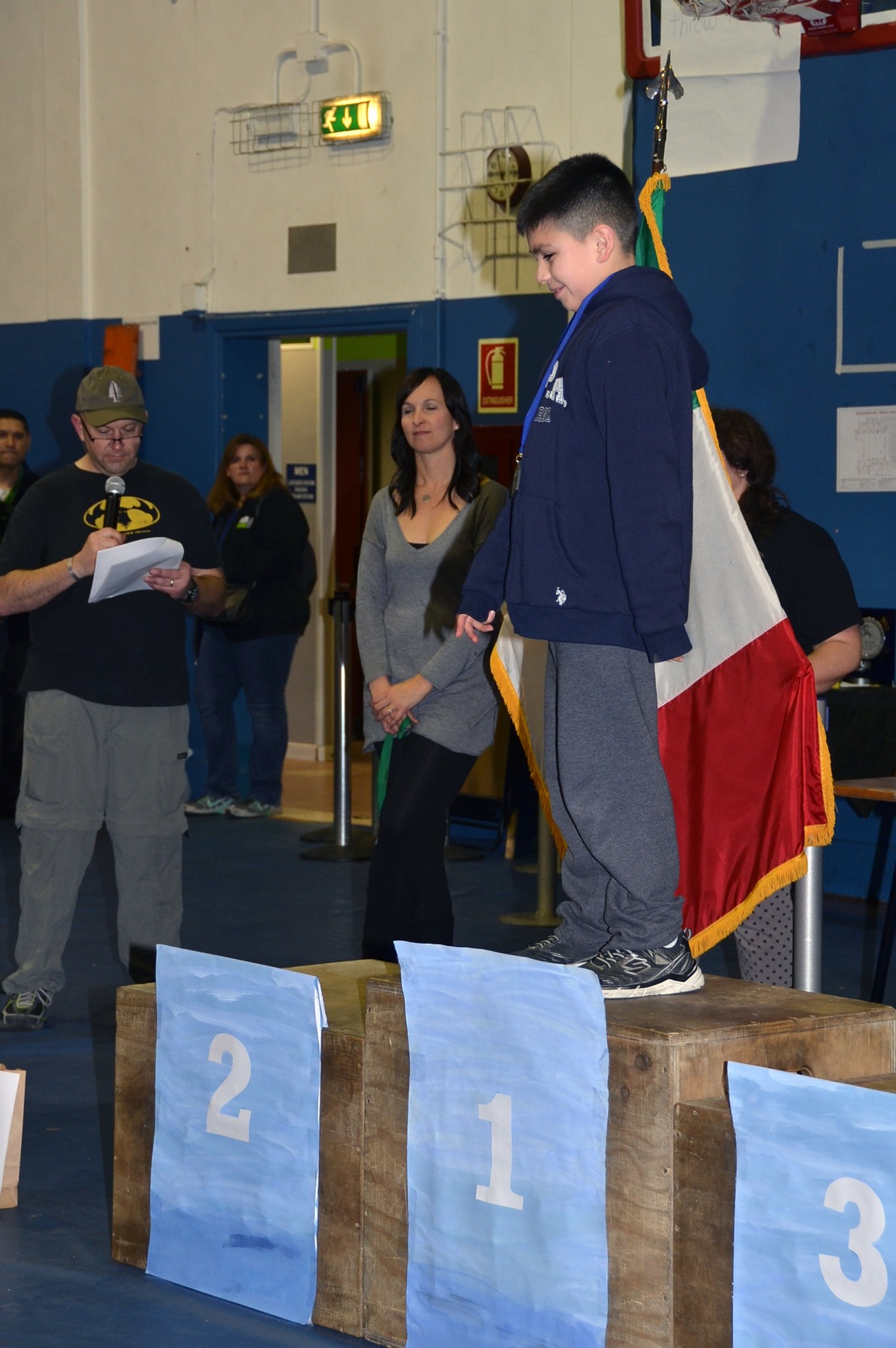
184 795 234 815
226 798 282 819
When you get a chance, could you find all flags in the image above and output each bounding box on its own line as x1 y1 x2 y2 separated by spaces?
486 163 836 962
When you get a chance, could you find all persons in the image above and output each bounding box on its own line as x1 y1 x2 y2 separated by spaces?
182 431 318 819
715 407 864 989
355 368 510 962
0 365 226 1032
455 152 709 998
0 408 42 537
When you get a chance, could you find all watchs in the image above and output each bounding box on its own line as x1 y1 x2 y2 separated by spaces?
180 577 199 603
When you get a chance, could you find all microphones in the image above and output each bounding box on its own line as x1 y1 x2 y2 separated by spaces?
102 476 125 529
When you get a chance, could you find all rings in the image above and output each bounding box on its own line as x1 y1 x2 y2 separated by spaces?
387 706 393 714
170 579 174 586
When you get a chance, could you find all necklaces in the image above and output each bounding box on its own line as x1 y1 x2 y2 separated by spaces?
414 482 447 503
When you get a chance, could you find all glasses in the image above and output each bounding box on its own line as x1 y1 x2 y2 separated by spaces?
79 415 145 445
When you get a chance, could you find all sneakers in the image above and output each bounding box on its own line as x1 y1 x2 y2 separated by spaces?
0 988 53 1031
511 933 599 967
578 928 704 998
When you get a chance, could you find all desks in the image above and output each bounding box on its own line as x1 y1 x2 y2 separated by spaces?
833 776 896 1003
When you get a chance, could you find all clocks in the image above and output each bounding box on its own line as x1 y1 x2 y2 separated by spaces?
487 146 531 210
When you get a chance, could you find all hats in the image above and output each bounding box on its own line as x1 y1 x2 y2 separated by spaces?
75 365 148 427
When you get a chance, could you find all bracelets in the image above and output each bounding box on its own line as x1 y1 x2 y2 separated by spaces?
67 557 82 584
